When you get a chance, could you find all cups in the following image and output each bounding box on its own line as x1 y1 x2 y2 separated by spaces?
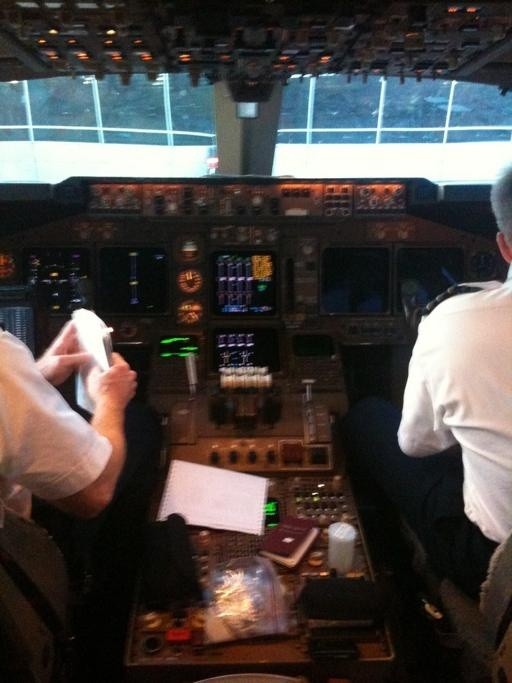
327 522 357 574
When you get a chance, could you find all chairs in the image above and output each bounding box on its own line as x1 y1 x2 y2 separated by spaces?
0 498 93 682
427 519 512 679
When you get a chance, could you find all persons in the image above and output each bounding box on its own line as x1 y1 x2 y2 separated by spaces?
340 160 512 598
0 320 140 522
